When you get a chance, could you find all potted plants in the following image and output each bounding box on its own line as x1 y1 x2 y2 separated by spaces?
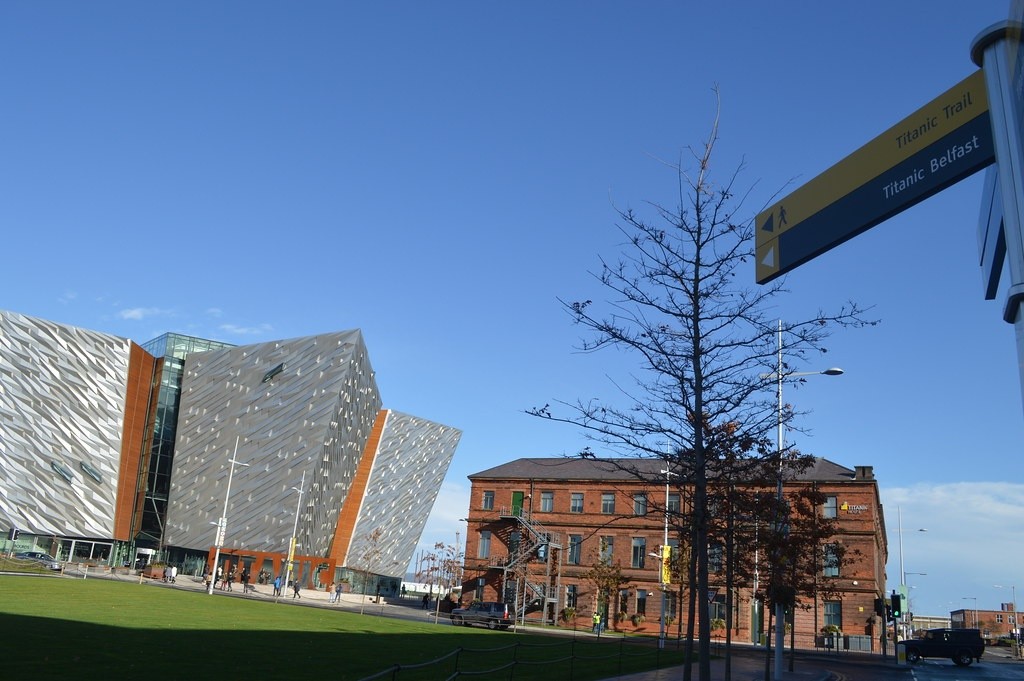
821 625 844 648
145 564 164 577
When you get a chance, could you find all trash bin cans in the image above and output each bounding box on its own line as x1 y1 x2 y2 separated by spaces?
829 632 844 650
859 635 871 651
849 635 860 650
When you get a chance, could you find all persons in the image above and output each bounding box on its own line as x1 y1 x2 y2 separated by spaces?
164 563 177 583
592 612 605 634
202 563 301 598
376 583 461 610
328 581 342 603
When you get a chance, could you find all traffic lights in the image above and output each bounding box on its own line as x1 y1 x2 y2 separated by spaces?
12 529 20 541
891 595 901 618
887 605 893 622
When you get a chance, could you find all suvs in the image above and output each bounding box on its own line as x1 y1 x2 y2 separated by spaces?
450 601 516 630
898 628 985 666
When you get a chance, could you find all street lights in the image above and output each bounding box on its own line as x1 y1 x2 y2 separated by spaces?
992 585 1019 655
899 528 927 640
961 597 978 629
648 552 665 648
948 602 961 628
775 367 844 681
938 605 948 628
283 487 302 599
904 572 928 639
208 461 250 593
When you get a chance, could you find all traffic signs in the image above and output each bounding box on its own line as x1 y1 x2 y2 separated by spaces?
755 0 1024 302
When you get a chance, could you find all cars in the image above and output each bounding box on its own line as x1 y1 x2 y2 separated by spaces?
15 552 65 570
